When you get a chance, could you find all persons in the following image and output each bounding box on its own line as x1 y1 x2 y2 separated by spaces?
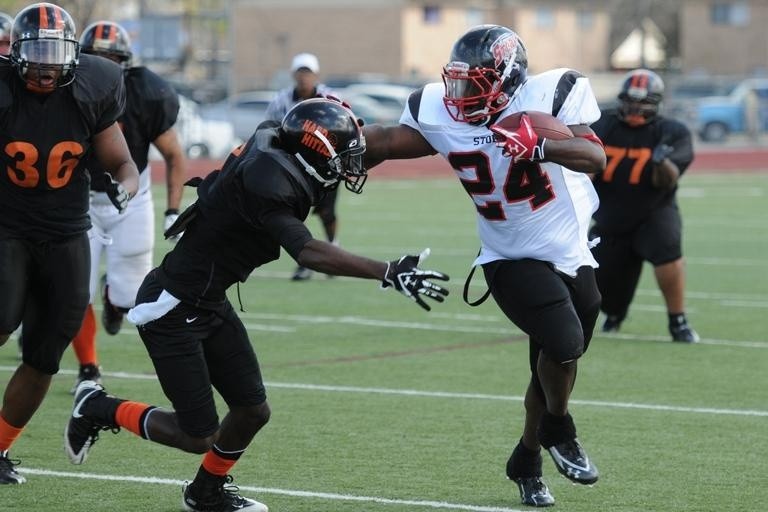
593 68 702 345
66 97 449 512
70 21 187 397
265 52 337 279
310 24 607 506
0 3 141 484
0 13 12 57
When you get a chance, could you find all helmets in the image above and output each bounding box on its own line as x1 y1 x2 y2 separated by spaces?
81 22 133 63
10 2 76 74
618 70 666 107
445 25 528 108
281 97 366 194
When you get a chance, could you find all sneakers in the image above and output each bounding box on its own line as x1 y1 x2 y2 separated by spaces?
292 260 314 282
536 410 601 486
180 474 269 512
68 364 104 394
0 448 27 486
505 435 556 506
603 314 627 333
668 315 702 345
65 379 106 466
99 274 123 337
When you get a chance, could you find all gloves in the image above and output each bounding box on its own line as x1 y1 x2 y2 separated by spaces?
315 92 364 129
488 111 546 164
103 171 130 216
163 208 184 241
650 144 674 166
380 244 453 313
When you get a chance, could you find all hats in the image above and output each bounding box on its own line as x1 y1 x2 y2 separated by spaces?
289 53 319 76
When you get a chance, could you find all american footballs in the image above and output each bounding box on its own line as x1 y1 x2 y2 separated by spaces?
493 112 574 141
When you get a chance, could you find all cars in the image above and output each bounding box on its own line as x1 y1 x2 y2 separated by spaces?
135 60 424 165
595 65 767 144
